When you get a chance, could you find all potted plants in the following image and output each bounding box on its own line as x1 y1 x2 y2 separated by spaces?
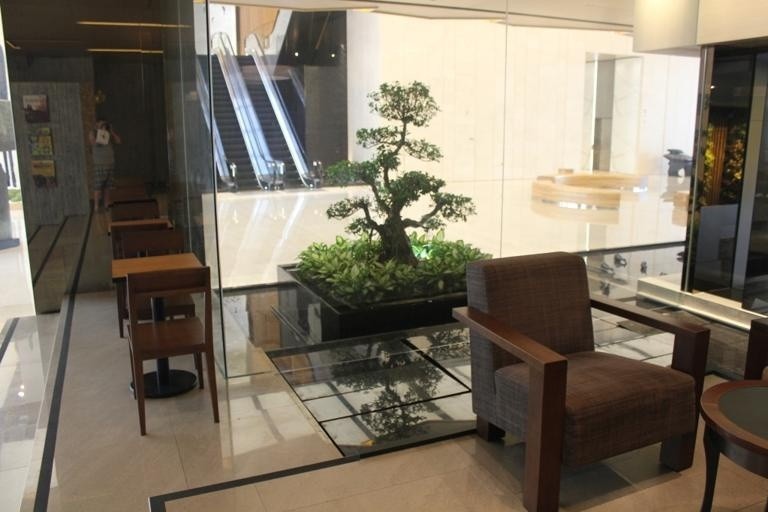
265 67 495 348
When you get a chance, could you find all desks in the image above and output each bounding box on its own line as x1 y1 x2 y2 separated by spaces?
691 376 768 511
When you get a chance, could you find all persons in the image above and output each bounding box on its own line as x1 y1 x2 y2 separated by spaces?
88 115 122 214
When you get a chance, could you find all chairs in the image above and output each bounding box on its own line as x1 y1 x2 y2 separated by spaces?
102 173 220 438
453 252 712 512
